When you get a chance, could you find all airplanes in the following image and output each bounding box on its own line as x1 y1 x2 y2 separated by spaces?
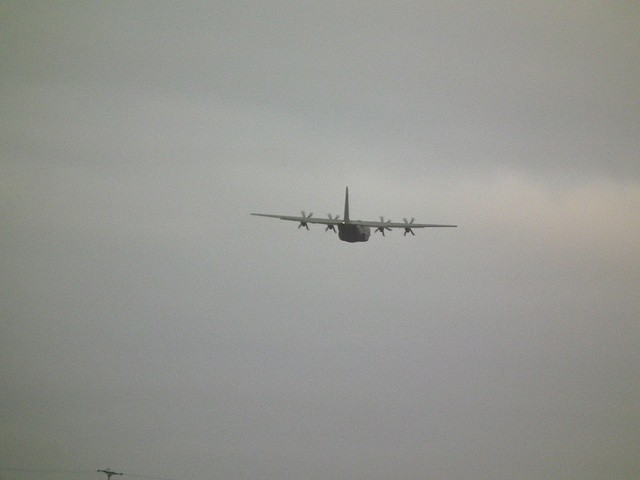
250 186 458 243
